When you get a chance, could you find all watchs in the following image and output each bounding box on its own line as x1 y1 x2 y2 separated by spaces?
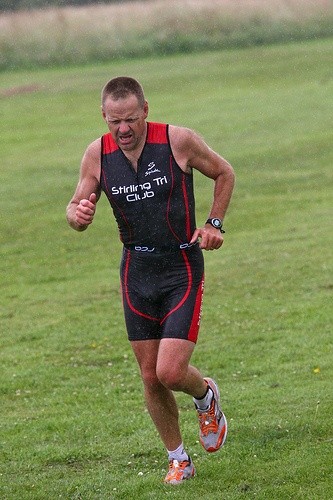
206 218 225 233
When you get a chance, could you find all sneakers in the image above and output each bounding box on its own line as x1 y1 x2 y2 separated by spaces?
194 377 227 452
163 455 195 485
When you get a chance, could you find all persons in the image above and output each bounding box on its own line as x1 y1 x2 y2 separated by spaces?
64 76 235 483
